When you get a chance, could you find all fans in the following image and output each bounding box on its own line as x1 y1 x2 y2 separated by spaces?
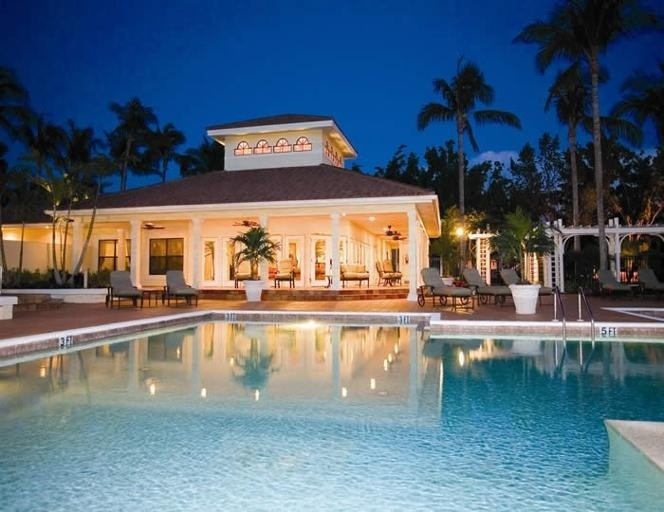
141 222 166 232
229 219 260 228
376 225 400 236
384 233 407 242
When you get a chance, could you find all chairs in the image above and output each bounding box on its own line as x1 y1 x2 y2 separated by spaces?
459 267 513 308
232 257 401 289
160 269 199 309
414 266 474 311
491 267 553 306
102 270 142 309
638 266 664 300
595 268 634 302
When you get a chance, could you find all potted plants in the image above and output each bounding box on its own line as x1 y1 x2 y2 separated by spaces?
228 224 283 303
224 322 284 395
486 204 562 316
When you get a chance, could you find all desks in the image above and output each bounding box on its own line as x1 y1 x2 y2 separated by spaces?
137 287 167 308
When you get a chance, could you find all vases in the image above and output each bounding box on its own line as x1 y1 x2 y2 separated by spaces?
510 340 541 357
